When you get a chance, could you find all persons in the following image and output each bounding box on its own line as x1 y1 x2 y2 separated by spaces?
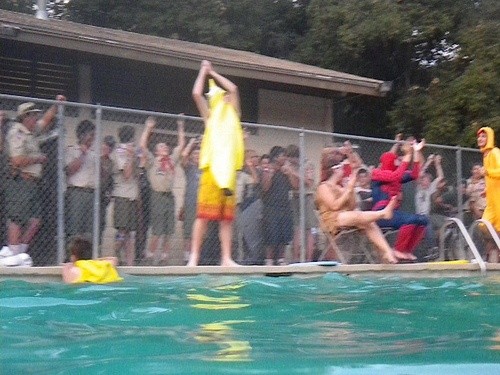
315 127 500 263
61 238 121 285
0 94 315 265
184 60 245 267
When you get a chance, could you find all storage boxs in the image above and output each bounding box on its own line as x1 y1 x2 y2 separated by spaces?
294 228 353 265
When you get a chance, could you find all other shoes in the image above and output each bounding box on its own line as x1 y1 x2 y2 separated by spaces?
263 258 274 265
278 258 288 265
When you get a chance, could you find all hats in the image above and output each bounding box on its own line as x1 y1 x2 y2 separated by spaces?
16 102 43 116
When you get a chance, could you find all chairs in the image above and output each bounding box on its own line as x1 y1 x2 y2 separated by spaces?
312 208 375 264
